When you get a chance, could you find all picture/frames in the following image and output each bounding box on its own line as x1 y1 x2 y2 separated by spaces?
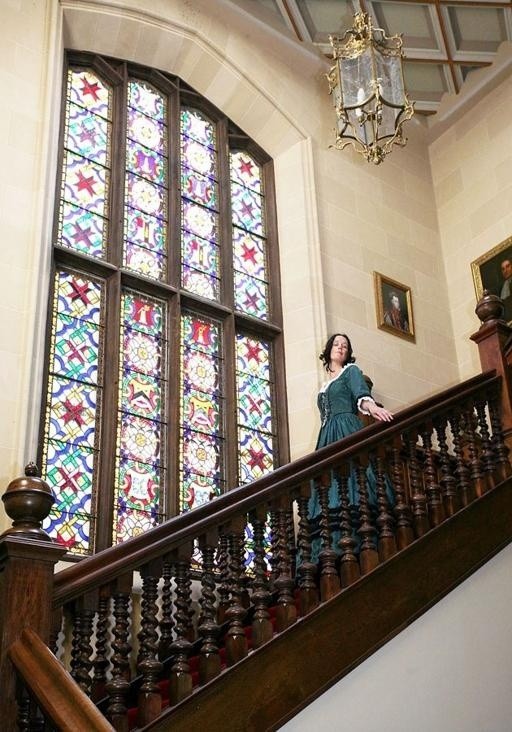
470 236 512 329
373 271 415 344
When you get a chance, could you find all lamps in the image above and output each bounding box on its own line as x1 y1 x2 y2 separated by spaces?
324 11 416 165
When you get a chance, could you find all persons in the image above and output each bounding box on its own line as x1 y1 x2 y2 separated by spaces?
500 260 512 318
295 334 395 578
384 292 408 331
